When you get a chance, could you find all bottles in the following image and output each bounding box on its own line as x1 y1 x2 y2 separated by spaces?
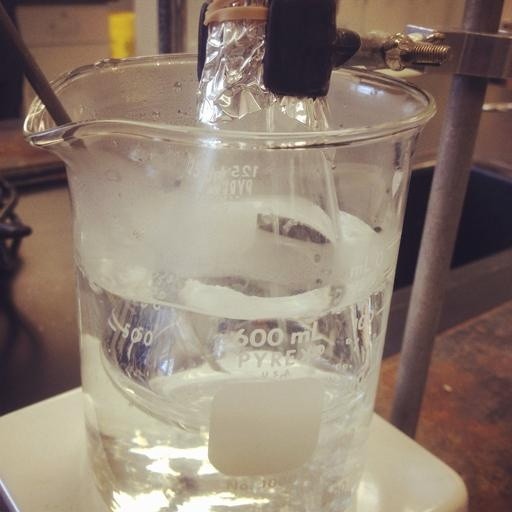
97 1 374 438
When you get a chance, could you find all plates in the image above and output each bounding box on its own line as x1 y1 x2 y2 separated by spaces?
1 346 467 512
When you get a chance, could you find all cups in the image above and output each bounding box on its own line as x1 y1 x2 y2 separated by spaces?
21 47 437 512
105 13 138 57
